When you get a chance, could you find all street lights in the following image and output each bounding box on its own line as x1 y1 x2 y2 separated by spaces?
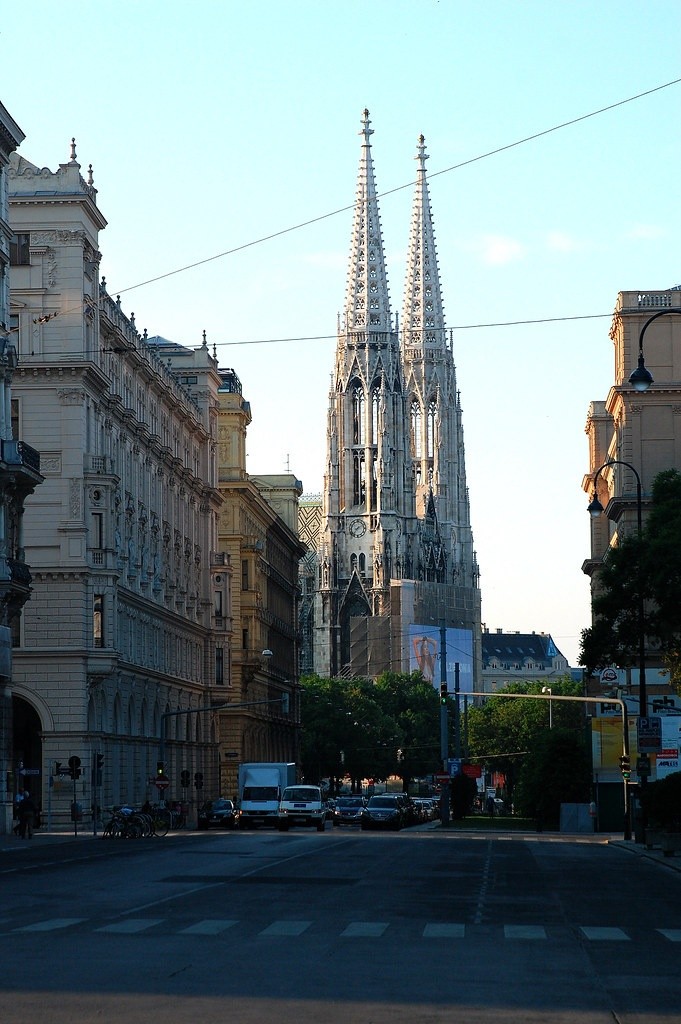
587 461 648 828
541 686 552 730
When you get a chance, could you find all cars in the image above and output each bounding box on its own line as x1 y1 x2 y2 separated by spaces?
325 795 452 825
198 796 236 830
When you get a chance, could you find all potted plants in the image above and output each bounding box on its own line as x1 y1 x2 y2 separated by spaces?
638 779 672 850
651 773 681 857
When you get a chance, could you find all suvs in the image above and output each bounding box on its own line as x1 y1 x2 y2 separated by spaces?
361 795 406 831
378 791 418 827
332 796 367 826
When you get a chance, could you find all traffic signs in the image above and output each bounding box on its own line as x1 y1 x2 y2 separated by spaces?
19 767 40 776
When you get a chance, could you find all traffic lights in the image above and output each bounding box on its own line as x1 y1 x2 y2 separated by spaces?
441 683 449 706
619 755 632 781
96 753 103 768
157 761 163 775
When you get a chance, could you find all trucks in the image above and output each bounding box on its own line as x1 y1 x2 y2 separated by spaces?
233 762 297 829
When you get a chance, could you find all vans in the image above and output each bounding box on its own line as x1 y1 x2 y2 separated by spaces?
276 784 328 832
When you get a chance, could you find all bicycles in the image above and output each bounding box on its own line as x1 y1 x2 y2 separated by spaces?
100 801 171 840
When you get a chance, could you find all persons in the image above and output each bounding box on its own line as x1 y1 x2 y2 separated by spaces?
484 796 497 819
232 796 238 810
12 787 38 840
413 636 437 685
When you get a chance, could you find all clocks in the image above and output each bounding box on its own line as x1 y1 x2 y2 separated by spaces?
349 519 366 538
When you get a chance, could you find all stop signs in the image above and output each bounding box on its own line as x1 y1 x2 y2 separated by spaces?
155 775 170 790
